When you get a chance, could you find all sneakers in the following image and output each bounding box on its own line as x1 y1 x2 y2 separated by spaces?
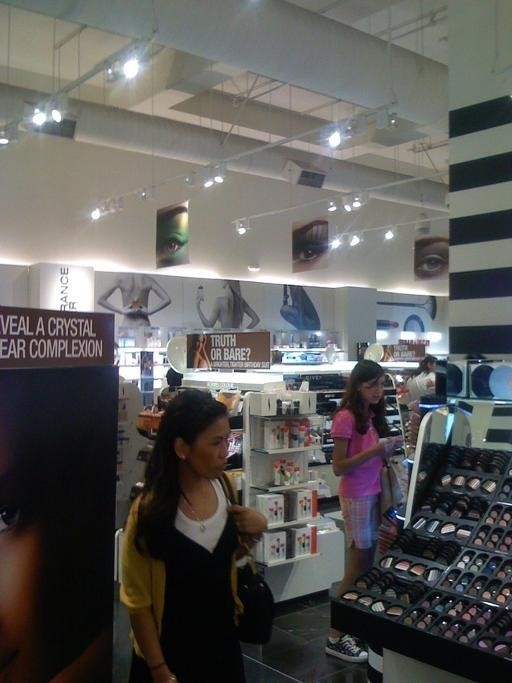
324 634 369 663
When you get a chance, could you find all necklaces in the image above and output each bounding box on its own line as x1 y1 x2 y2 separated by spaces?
176 479 211 533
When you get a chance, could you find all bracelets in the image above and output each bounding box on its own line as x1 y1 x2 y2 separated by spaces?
149 662 167 672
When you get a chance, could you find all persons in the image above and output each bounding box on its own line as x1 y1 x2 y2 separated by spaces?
323 360 397 662
1 391 112 682
97 272 171 328
280 285 320 329
194 280 259 329
414 233 450 282
118 386 274 683
291 216 328 273
398 352 439 474
190 334 211 369
155 204 189 269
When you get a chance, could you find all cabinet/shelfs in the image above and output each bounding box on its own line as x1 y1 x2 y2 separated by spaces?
118 344 436 604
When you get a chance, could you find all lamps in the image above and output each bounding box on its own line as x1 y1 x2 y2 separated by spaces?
204 162 224 188
232 217 247 236
1 91 68 149
327 191 367 213
87 196 119 224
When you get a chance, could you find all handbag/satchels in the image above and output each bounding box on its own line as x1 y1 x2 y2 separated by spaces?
379 456 404 522
229 560 275 646
